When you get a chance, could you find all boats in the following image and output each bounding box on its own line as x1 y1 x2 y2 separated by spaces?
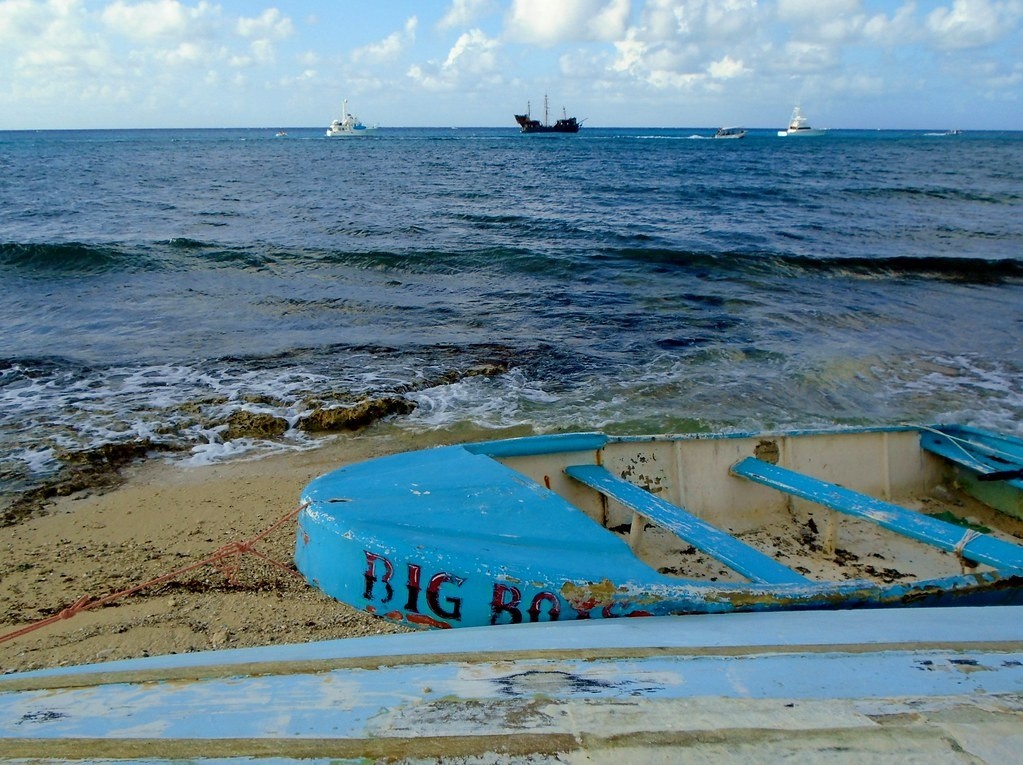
778 107 827 136
276 133 287 137
326 98 369 136
514 94 590 133
714 128 746 139
293 422 1023 632
